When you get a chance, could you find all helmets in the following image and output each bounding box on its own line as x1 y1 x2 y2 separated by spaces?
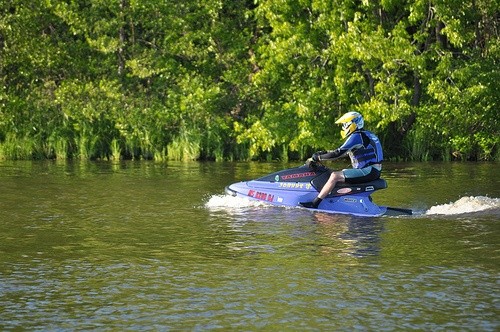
335 112 364 139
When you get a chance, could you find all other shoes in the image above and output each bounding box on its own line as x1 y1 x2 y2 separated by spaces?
298 201 318 209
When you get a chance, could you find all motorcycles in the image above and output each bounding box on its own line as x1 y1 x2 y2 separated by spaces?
225 152 426 218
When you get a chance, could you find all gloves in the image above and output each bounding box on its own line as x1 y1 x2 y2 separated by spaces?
312 153 319 161
317 150 327 156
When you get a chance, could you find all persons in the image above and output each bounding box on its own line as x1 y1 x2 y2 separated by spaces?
298 112 384 209
